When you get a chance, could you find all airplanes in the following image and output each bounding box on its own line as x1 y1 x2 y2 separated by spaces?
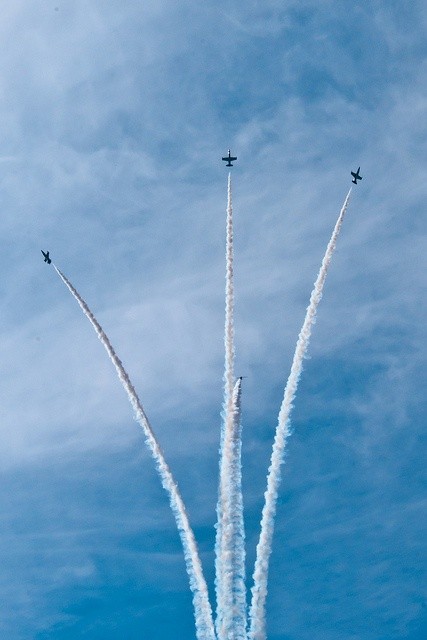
221 149 238 167
40 250 52 264
350 166 362 184
